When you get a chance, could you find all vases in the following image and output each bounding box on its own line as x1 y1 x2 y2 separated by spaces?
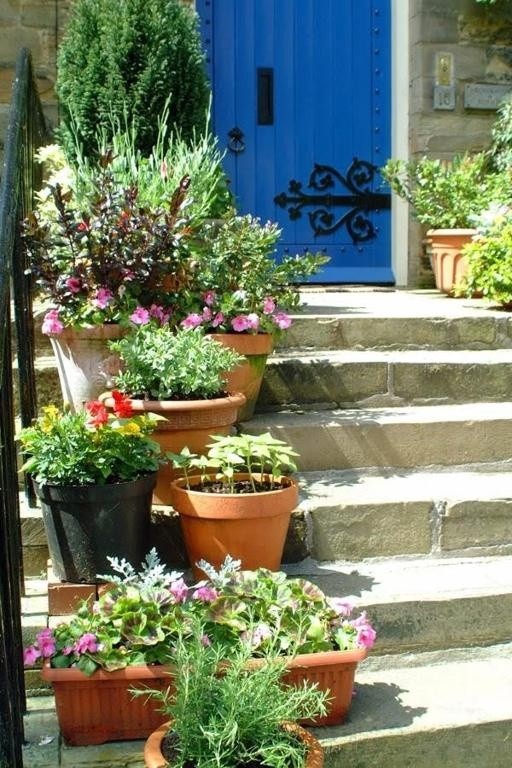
500 298 511 312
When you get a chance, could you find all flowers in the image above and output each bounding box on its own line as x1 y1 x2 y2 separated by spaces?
445 195 512 304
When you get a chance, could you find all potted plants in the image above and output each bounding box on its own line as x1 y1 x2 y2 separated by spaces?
15 389 164 585
375 148 501 300
47 0 214 185
30 94 330 422
131 603 335 768
166 433 305 573
102 326 249 507
22 569 381 748
